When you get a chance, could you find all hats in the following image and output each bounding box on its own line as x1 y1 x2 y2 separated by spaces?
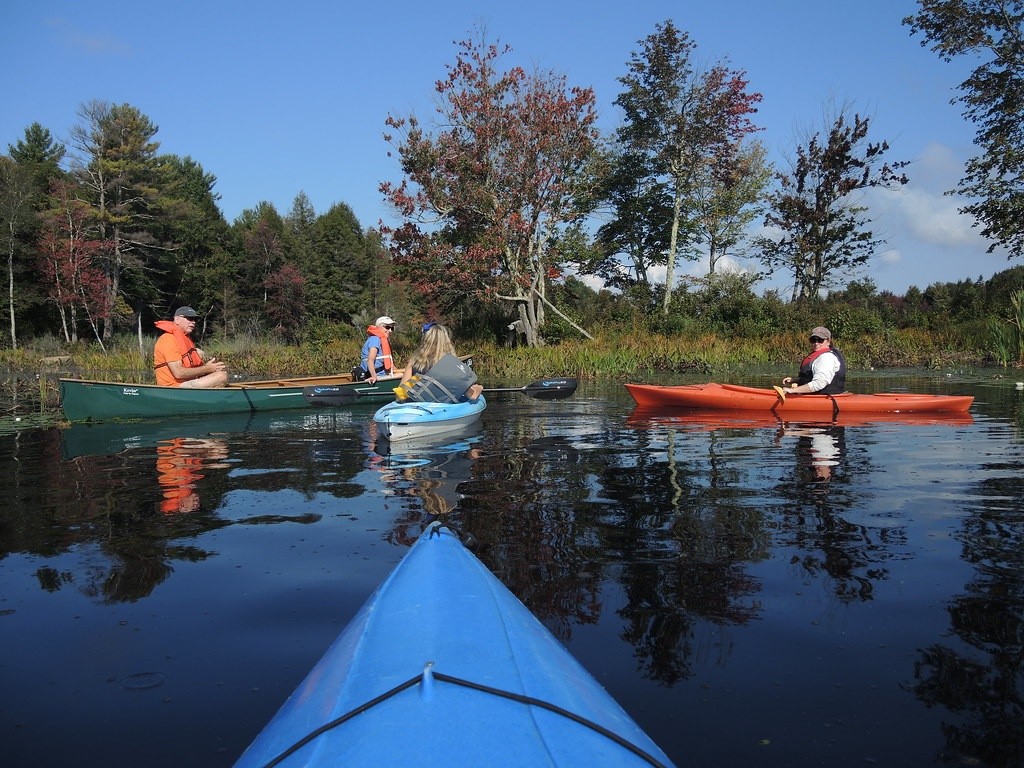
376 316 398 326
808 326 831 340
174 306 203 323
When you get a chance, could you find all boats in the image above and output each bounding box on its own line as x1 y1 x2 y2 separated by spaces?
623 382 975 413
371 393 488 442
58 354 476 423
61 401 392 460
623 407 975 426
372 420 487 469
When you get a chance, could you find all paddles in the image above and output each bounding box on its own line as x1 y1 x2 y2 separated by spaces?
299 375 582 409
771 385 792 403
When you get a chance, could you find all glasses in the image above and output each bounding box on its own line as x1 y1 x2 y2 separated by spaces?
810 337 828 343
177 315 198 324
378 325 394 332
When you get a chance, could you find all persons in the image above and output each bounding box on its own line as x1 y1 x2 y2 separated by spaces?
155 438 230 511
782 327 845 395
154 307 228 389
360 316 398 384
775 424 841 485
396 323 484 404
